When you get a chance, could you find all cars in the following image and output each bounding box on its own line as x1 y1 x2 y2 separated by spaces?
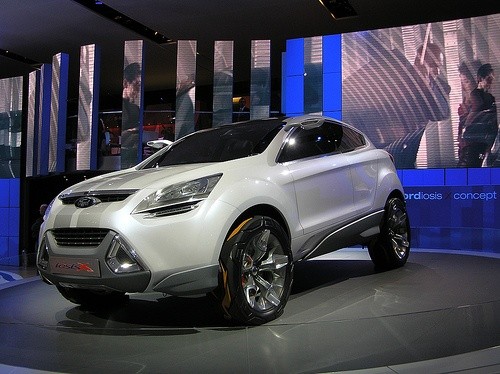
38 116 412 322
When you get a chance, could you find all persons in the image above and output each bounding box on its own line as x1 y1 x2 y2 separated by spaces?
121 62 141 167
383 44 500 170
